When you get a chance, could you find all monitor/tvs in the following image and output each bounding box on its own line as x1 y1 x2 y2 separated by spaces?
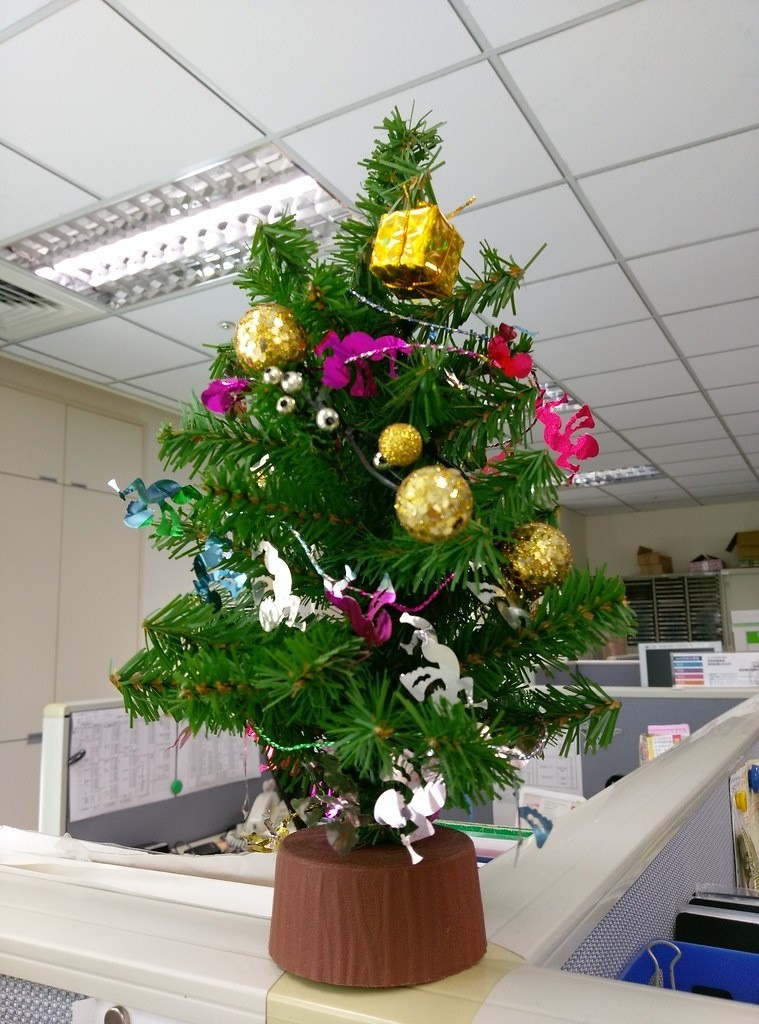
638 642 722 687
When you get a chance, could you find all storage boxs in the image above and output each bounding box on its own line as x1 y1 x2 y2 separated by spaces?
636 546 673 565
739 559 759 567
689 554 723 572
726 530 759 560
639 565 673 575
